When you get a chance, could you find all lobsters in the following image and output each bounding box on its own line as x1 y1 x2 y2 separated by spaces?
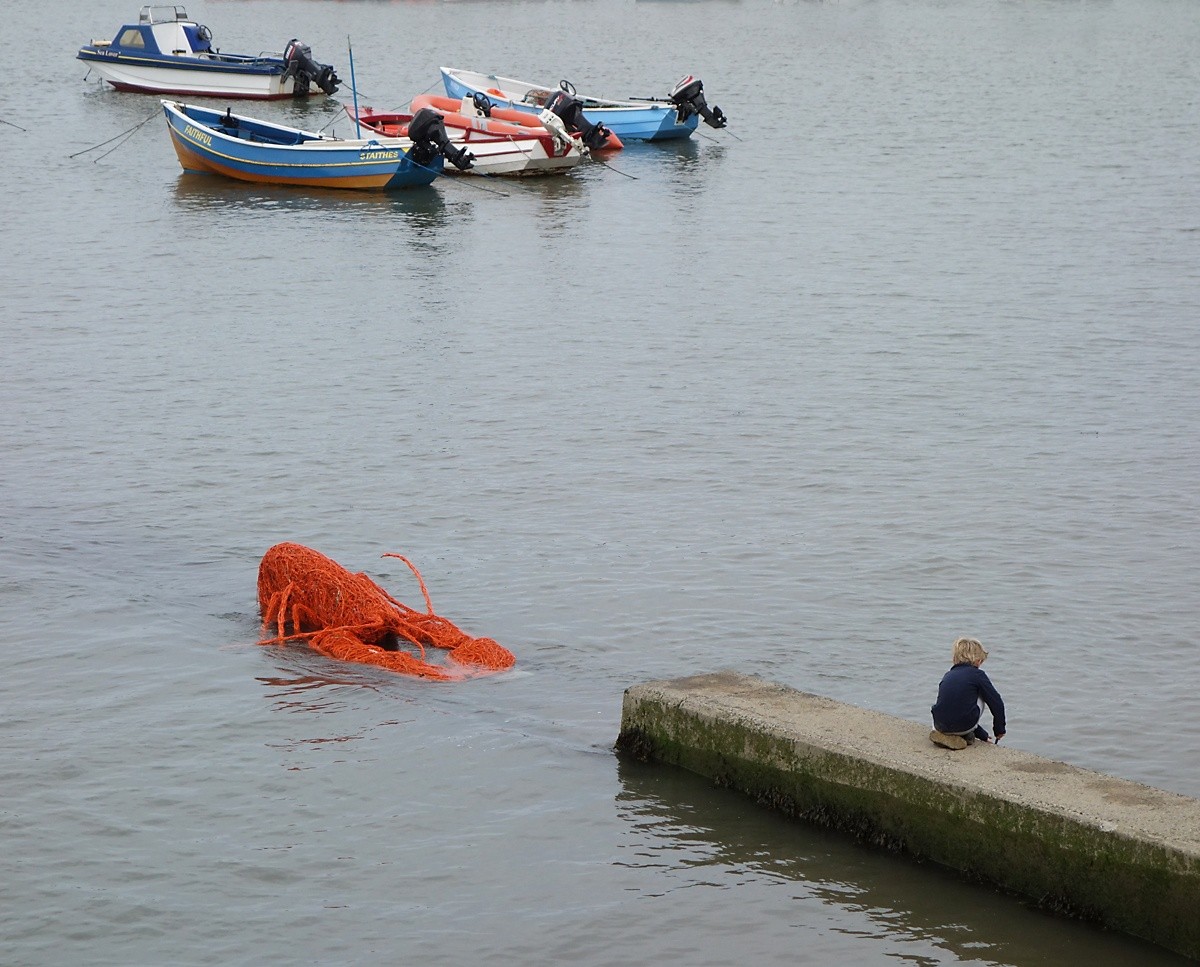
256 542 516 682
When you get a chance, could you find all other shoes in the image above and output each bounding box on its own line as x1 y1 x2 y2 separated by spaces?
929 730 967 750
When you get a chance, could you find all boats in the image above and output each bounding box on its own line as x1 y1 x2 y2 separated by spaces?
159 98 478 190
74 5 342 101
406 79 625 151
341 102 595 176
438 65 729 140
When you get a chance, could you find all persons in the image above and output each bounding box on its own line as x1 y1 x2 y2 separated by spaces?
929 636 1006 750
